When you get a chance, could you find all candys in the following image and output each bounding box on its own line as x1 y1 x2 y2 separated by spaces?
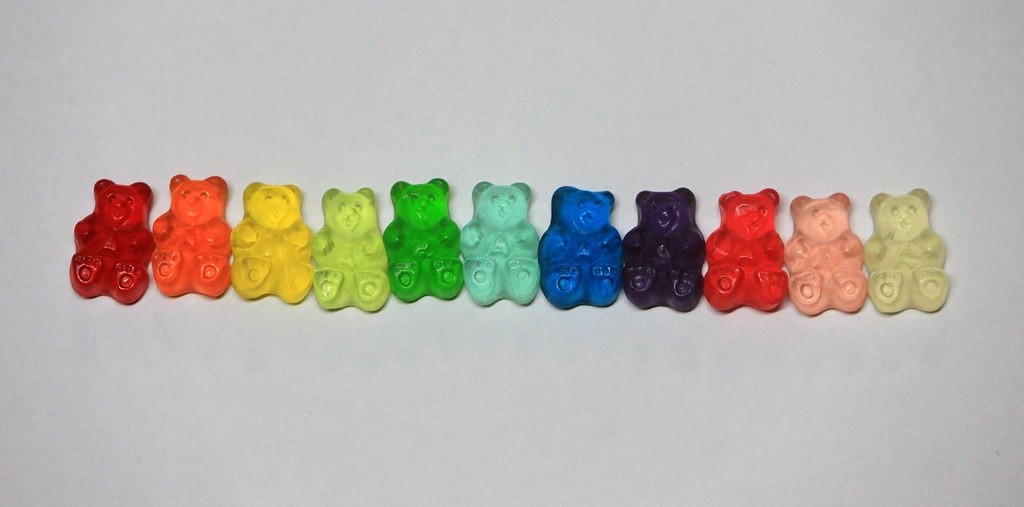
69 176 951 316
